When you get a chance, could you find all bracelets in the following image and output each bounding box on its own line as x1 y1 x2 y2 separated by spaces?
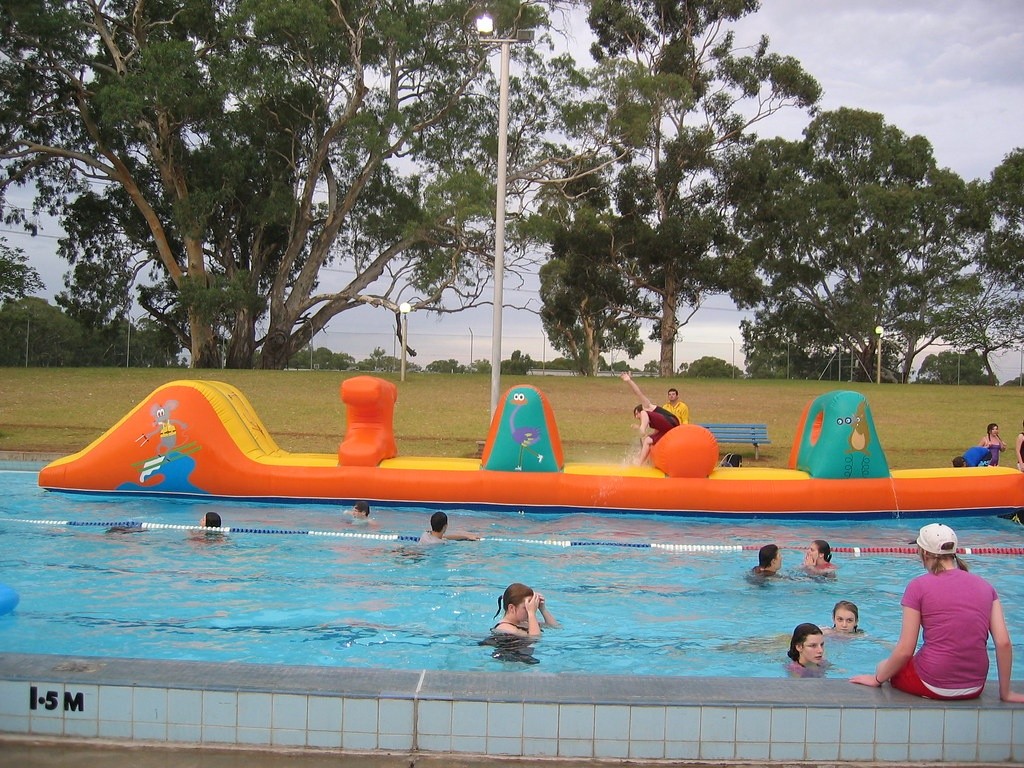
876 676 882 684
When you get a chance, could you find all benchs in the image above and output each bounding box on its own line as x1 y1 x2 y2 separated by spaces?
699 423 772 461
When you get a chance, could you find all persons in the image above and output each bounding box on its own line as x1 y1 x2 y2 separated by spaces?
953 446 991 467
422 512 479 540
1016 420 1024 472
805 540 836 570
619 371 680 465
788 623 824 668
753 545 781 573
494 583 555 636
832 601 859 632
979 423 1007 466
200 512 221 527
663 388 688 424
352 501 370 517
848 524 1024 703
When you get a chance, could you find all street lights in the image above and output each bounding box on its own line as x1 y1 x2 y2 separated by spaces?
875 325 884 385
399 302 411 383
473 7 534 464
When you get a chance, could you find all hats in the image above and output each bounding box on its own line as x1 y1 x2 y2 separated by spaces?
909 523 958 554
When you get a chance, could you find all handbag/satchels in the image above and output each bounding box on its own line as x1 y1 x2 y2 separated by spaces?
725 454 742 467
